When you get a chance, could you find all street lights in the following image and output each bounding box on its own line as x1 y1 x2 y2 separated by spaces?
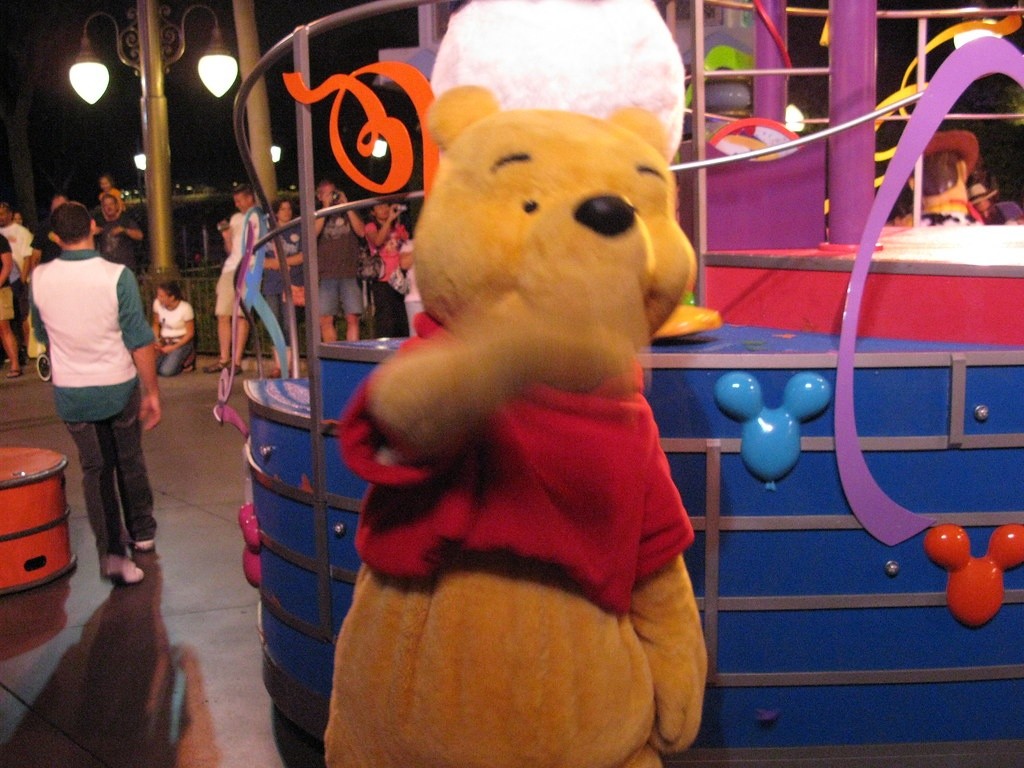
67 0 238 281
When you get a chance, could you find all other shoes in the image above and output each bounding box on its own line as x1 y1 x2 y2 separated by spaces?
132 536 156 552
100 554 144 585
226 363 242 376
202 360 231 374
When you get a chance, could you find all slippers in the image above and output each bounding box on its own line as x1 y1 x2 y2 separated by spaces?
6 367 22 378
183 358 195 373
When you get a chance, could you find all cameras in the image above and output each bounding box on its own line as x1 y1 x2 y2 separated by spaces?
331 193 340 201
397 205 407 211
218 223 229 230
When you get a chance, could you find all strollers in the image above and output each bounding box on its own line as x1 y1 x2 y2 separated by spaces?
30 244 60 382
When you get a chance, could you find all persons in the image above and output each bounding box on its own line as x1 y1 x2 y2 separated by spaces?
0 175 194 378
201 186 425 376
968 183 1006 225
28 201 161 587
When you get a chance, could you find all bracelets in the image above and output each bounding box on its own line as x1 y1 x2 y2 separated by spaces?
123 227 127 233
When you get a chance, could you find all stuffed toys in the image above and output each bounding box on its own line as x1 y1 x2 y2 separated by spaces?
324 89 708 768
